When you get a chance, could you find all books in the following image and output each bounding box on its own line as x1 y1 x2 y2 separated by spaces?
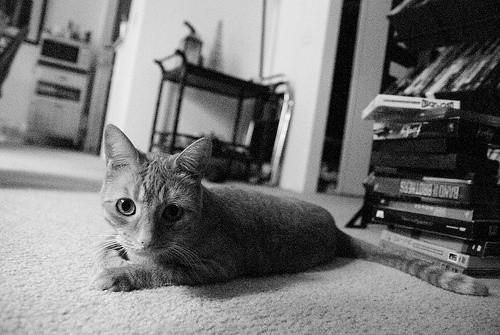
360 40 500 279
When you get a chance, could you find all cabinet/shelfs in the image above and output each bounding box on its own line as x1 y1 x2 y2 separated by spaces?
148 49 285 183
24 60 90 151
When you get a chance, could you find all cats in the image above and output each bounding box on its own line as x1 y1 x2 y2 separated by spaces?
87 124 490 297
